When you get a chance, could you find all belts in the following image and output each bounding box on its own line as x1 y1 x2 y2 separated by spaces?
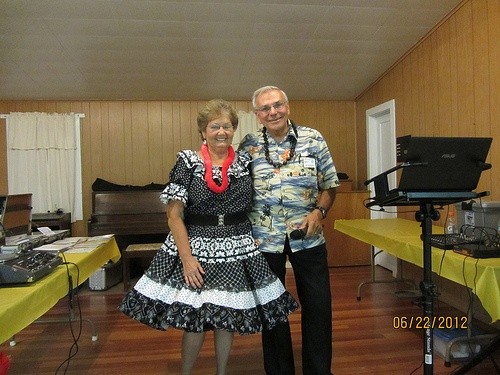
184 212 247 227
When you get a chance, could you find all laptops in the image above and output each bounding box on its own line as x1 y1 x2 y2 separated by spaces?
370 137 493 199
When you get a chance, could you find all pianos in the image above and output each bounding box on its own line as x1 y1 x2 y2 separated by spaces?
85 187 171 250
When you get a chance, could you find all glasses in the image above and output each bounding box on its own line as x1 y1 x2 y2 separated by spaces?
205 123 232 131
260 102 286 113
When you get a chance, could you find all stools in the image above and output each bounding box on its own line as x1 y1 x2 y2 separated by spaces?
123 241 165 290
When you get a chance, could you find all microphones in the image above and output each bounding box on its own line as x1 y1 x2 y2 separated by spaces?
289 223 325 239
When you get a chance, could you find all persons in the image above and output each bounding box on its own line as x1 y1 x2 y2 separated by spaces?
118 99 300 374
238 86 339 375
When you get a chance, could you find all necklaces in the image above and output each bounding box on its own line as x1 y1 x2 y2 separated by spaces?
263 119 297 167
201 143 235 193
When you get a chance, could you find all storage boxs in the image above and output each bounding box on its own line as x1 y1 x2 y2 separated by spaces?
340 179 353 191
454 201 500 239
432 316 480 363
89 260 124 292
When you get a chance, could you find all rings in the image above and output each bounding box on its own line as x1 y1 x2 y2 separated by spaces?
315 232 317 234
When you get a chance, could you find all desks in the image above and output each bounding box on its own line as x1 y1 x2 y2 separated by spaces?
0 233 122 346
32 213 71 235
334 216 500 375
317 190 373 268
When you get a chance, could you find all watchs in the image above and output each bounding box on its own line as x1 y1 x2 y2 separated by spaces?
315 207 326 218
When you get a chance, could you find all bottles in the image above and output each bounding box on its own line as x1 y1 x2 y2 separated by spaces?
445 211 456 234
